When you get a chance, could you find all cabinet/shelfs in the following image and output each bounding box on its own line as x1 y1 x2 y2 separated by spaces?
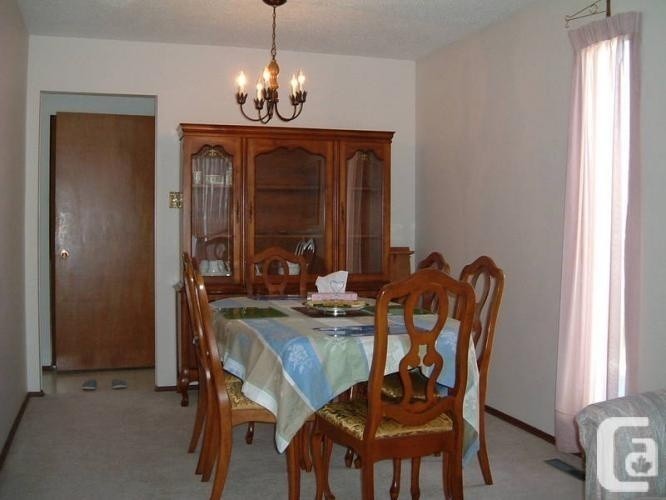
175 121 397 407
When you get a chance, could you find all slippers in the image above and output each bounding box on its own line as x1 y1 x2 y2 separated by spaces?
81 379 96 390
112 379 128 389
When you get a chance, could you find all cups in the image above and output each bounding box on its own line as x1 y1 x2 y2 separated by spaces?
192 171 202 184
198 259 227 273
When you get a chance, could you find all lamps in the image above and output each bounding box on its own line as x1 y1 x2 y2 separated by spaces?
234 0 309 126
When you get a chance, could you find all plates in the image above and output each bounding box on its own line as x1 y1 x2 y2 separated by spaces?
305 300 367 314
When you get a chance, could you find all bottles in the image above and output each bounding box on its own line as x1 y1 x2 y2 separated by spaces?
226 167 233 185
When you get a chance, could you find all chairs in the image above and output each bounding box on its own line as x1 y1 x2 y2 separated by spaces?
180 245 316 498
402 251 452 312
312 266 475 498
352 255 507 486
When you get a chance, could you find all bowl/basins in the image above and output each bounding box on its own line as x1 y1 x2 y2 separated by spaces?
277 259 301 276
205 174 224 185
